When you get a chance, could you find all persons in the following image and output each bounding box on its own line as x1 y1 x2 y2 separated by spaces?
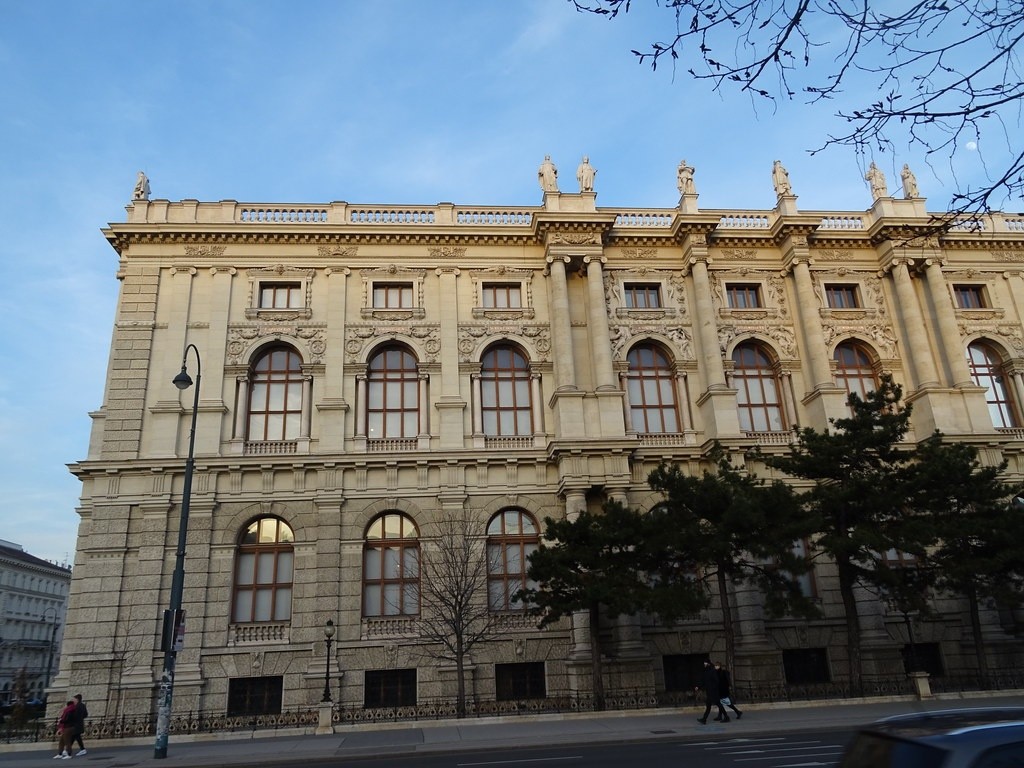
697 657 742 724
53 694 88 759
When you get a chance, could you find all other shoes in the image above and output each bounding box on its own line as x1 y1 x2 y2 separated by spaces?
697 718 707 725
713 716 722 721
719 716 731 724
736 711 743 720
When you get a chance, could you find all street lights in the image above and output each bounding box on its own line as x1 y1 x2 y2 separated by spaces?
40 607 57 719
154 345 202 759
315 619 336 735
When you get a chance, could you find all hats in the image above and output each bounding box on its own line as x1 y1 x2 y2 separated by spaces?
73 694 82 700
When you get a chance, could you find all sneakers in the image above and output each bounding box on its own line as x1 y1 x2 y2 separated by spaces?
75 749 87 756
62 750 68 756
62 754 72 760
53 754 64 759
